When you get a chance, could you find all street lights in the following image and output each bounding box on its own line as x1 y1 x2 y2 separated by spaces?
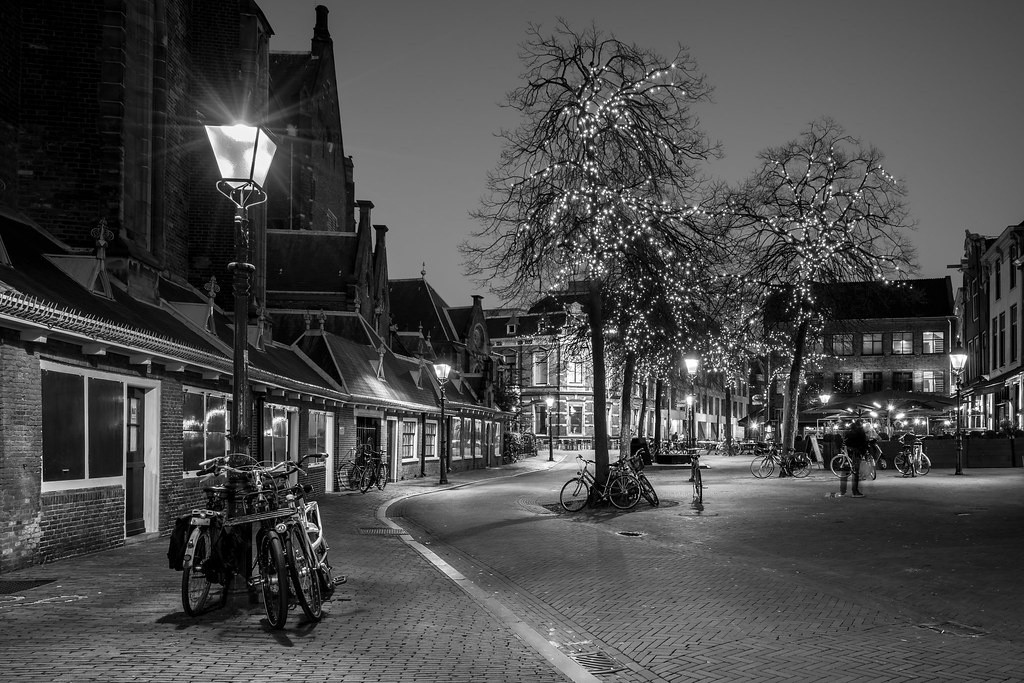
686 394 696 454
203 102 283 612
543 391 556 461
947 337 969 476
819 385 833 438
432 356 454 485
683 343 702 458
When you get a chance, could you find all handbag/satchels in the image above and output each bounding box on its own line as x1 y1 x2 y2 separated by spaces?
201 518 240 582
167 514 194 571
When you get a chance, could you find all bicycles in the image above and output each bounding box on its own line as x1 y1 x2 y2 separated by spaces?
560 454 643 513
645 434 703 460
620 447 659 507
716 436 816 479
166 452 347 631
830 442 876 481
684 446 708 504
338 444 391 494
893 431 934 477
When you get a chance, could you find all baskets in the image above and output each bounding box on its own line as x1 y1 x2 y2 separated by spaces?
627 454 644 474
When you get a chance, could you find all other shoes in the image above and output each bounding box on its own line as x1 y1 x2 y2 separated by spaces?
841 491 847 495
852 492 864 497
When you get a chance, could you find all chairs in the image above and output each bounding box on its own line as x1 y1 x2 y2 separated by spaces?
539 437 718 451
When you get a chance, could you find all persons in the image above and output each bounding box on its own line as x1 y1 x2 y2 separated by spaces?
837 420 865 498
671 432 678 442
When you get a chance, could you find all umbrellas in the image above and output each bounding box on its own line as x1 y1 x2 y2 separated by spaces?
802 386 970 432
